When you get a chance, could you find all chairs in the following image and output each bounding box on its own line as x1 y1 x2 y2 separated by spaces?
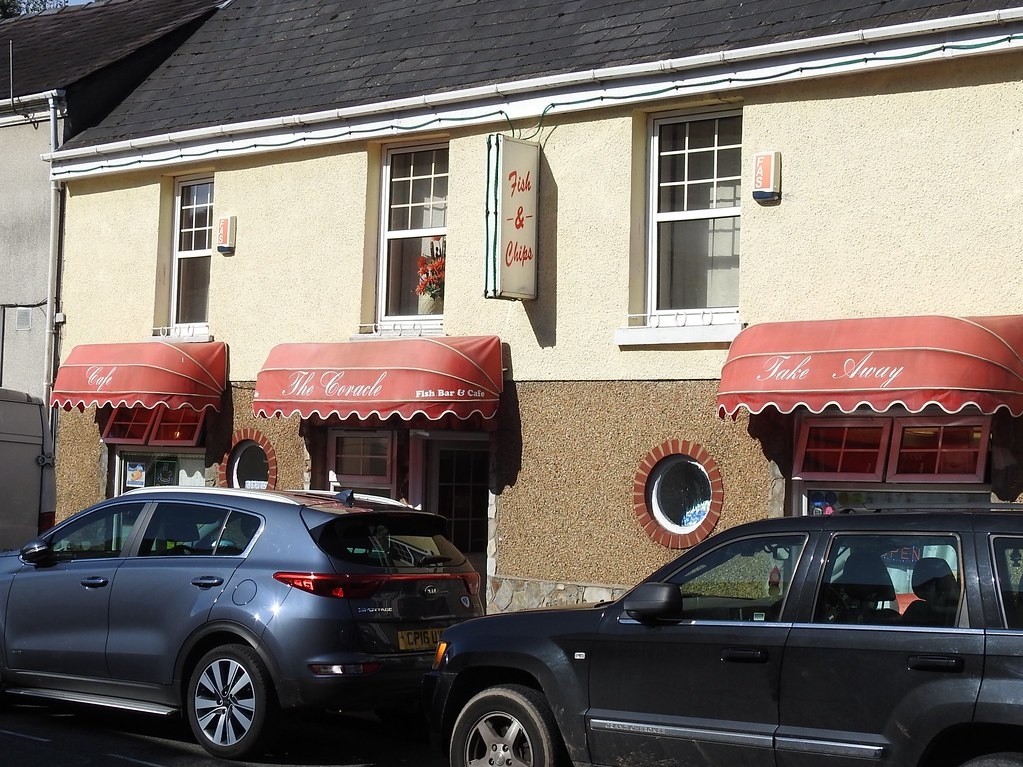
162 511 201 557
897 558 957 627
826 553 903 626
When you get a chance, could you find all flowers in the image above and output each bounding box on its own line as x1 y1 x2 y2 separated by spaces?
416 236 446 299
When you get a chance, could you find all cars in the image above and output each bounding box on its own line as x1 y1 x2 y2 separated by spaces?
0 489 488 761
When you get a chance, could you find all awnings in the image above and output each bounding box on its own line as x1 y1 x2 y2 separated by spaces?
49 342 227 414
253 334 503 421
715 314 1023 421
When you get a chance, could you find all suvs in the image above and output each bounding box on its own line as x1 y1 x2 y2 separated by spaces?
430 503 1023 767
